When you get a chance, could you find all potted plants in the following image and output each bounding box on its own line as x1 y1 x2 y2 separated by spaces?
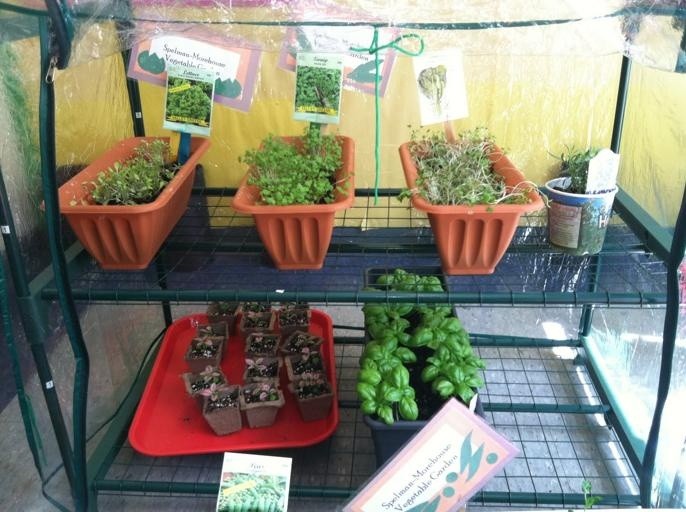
543 143 618 257
230 132 356 271
357 266 478 469
38 131 209 271
398 116 546 274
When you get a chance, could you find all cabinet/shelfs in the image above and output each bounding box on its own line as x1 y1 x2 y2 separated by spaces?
0 0 686 509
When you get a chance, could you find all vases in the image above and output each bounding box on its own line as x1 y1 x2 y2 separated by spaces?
187 308 333 434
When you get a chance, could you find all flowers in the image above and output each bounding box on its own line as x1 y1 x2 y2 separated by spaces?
190 302 319 403
